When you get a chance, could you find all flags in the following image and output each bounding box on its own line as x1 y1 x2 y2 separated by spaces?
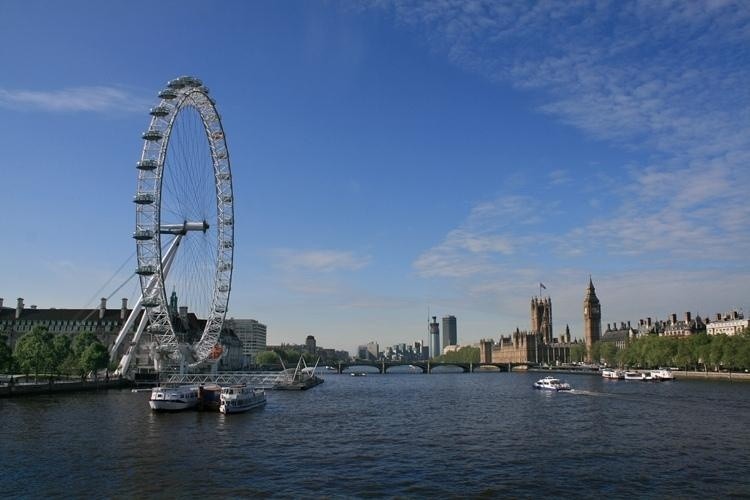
540 283 546 290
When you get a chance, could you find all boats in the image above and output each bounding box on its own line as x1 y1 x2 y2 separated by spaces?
219 383 266 415
349 371 367 376
532 375 571 392
602 369 675 381
149 384 200 410
